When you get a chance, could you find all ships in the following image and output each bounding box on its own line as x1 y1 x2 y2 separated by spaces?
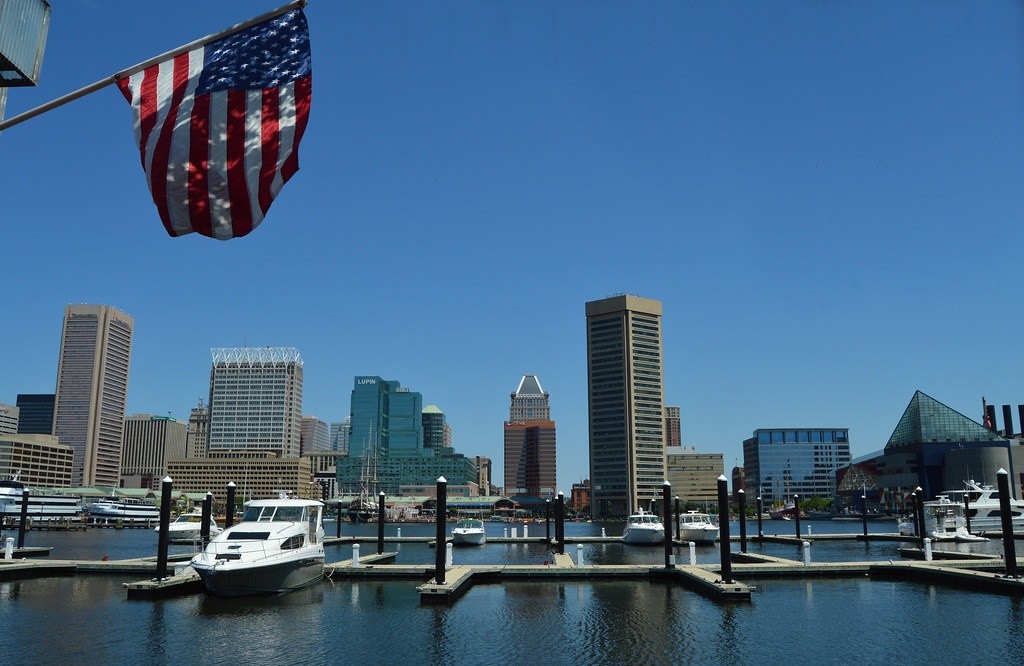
0 470 83 523
87 483 160 523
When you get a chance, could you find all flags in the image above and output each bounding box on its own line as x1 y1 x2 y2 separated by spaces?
116 11 312 238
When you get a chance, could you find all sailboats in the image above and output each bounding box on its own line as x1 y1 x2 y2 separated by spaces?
768 459 811 519
347 420 387 522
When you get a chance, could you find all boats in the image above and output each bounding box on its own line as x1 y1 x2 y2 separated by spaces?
921 496 986 543
155 513 223 544
623 507 664 544
675 511 719 544
896 478 1024 536
417 509 436 523
368 517 416 523
451 518 487 546
187 498 326 600
